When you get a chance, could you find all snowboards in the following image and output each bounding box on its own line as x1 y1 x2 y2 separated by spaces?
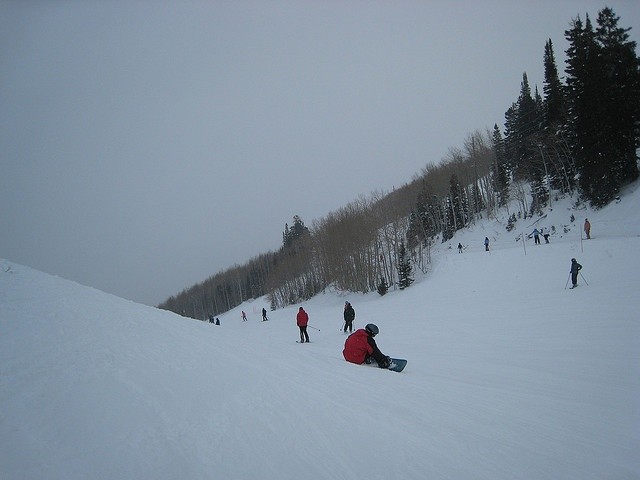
364 358 407 372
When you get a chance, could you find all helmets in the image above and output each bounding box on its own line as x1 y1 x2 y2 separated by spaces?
365 323 380 336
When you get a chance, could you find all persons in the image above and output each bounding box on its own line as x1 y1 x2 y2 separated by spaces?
215 318 220 325
241 311 248 322
342 324 398 369
571 258 582 287
540 227 550 243
296 307 309 343
344 301 355 332
528 228 542 244
484 237 489 251
457 243 463 253
261 308 268 321
584 218 591 239
209 315 214 323
